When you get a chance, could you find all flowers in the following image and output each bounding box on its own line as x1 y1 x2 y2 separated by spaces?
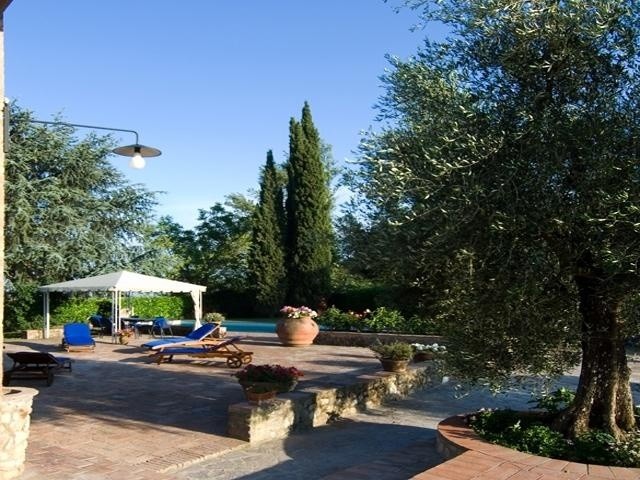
231 363 303 382
281 305 318 319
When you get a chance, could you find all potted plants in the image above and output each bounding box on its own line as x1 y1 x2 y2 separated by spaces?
371 338 416 373
417 349 434 362
244 385 279 406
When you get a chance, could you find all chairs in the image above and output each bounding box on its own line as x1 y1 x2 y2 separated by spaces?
3 315 252 386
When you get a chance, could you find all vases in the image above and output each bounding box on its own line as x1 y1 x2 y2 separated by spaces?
241 382 298 393
275 317 319 346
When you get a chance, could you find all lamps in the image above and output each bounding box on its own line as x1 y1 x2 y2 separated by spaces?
4 103 161 169
